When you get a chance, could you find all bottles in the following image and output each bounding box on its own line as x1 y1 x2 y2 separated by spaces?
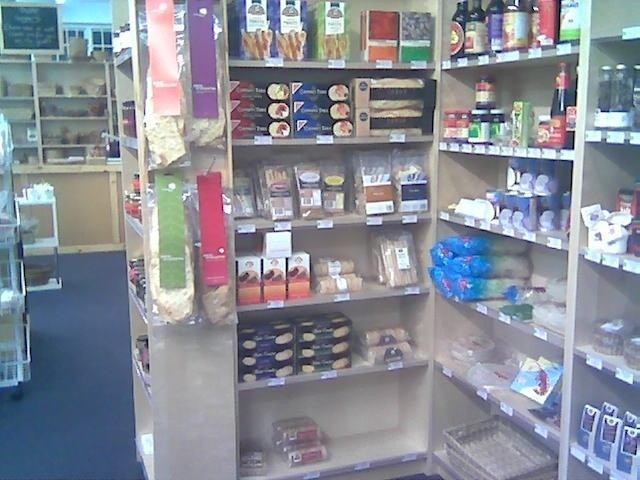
113 31 120 56
560 0 580 46
125 23 132 49
441 107 457 141
530 1 539 46
455 107 471 140
129 258 146 304
598 66 612 112
485 0 504 52
490 109 505 141
122 100 137 138
501 0 532 52
539 1 558 48
451 0 468 59
475 75 498 108
572 66 579 150
464 0 485 56
125 174 142 223
467 109 491 144
136 335 149 373
612 63 627 111
551 61 570 148
633 64 640 109
119 25 126 51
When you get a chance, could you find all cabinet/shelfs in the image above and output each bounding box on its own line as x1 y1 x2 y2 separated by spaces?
2 52 127 255
0 122 32 399
114 0 440 479
432 1 640 480
